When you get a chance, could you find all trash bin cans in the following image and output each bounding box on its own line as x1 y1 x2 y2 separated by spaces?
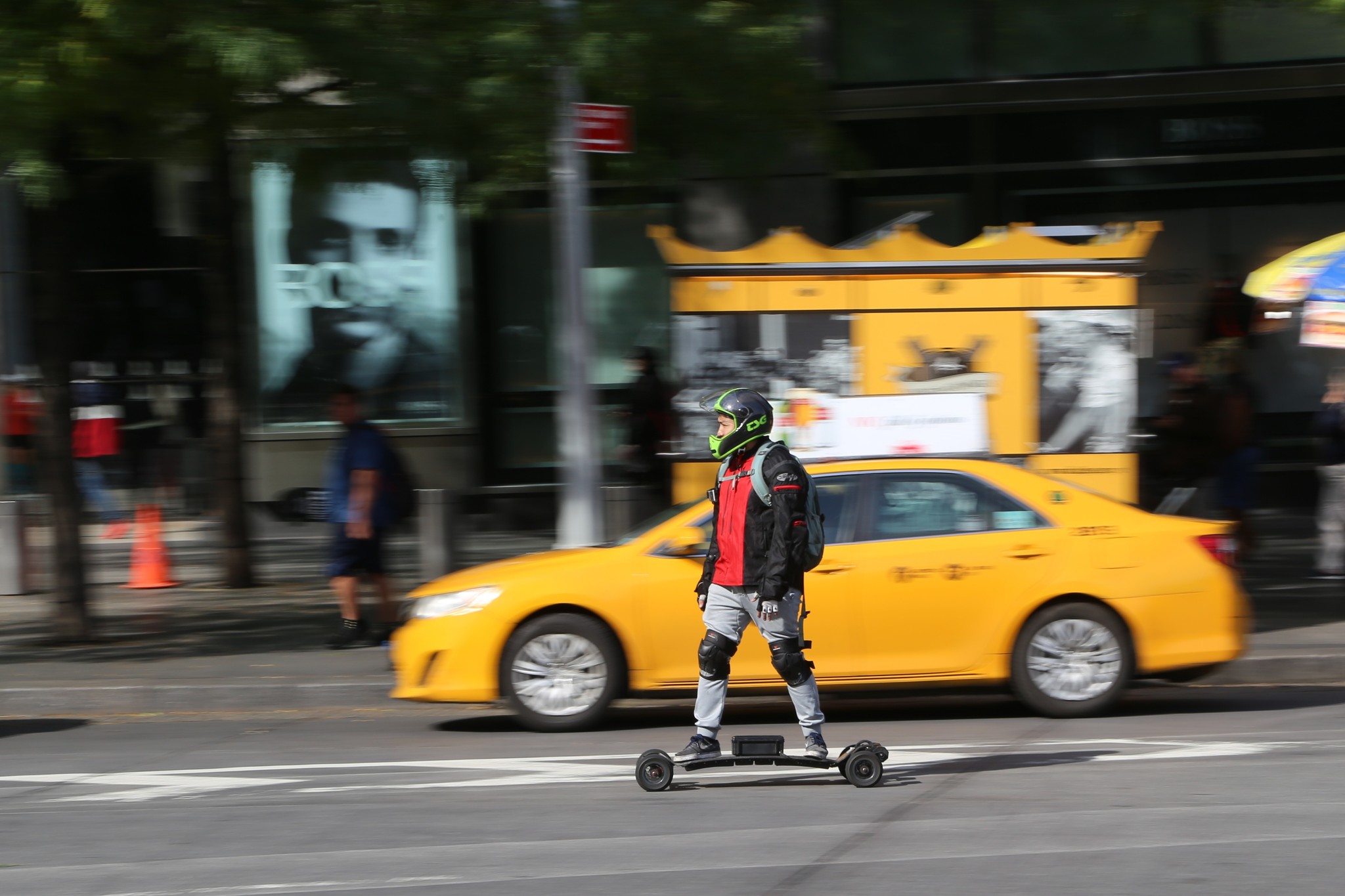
0 496 56 594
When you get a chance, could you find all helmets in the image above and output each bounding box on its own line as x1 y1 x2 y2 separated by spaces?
708 388 773 460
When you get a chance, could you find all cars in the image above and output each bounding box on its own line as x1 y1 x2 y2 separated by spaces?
386 451 1261 734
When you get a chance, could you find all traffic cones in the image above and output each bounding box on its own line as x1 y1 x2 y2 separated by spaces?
119 498 185 589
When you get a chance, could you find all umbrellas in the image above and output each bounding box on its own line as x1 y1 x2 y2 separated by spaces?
1242 231 1345 306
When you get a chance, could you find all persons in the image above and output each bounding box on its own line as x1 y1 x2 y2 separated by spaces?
323 383 400 647
0 373 132 540
619 345 679 530
675 388 829 761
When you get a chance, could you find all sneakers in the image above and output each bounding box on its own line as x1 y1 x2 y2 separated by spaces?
804 733 828 759
673 734 722 762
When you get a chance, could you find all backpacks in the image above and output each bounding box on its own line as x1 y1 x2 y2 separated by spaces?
718 443 826 572
